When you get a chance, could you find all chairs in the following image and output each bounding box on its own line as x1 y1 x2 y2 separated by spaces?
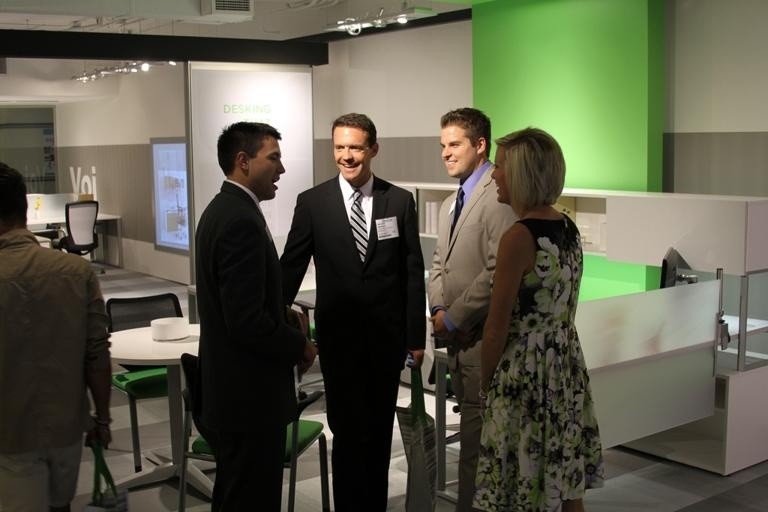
107 292 186 472
178 352 329 512
58 200 105 275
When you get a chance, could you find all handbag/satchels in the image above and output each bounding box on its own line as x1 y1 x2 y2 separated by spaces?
394 402 437 511
86 489 128 512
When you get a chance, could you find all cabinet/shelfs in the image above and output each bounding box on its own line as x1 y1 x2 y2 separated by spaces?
390 180 768 277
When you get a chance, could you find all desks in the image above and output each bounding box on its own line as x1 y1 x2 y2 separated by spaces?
25 192 124 268
108 324 217 503
434 267 768 503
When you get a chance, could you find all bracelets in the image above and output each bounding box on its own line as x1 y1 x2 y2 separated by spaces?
92 416 113 425
478 389 487 400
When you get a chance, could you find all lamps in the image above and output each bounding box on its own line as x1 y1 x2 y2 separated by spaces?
323 1 437 37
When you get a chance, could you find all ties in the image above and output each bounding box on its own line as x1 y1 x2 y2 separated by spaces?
448 187 465 244
350 190 369 263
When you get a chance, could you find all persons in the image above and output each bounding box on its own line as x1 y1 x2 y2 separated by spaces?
277 112 428 512
427 108 518 512
0 162 113 511
193 121 323 512
471 128 604 512
